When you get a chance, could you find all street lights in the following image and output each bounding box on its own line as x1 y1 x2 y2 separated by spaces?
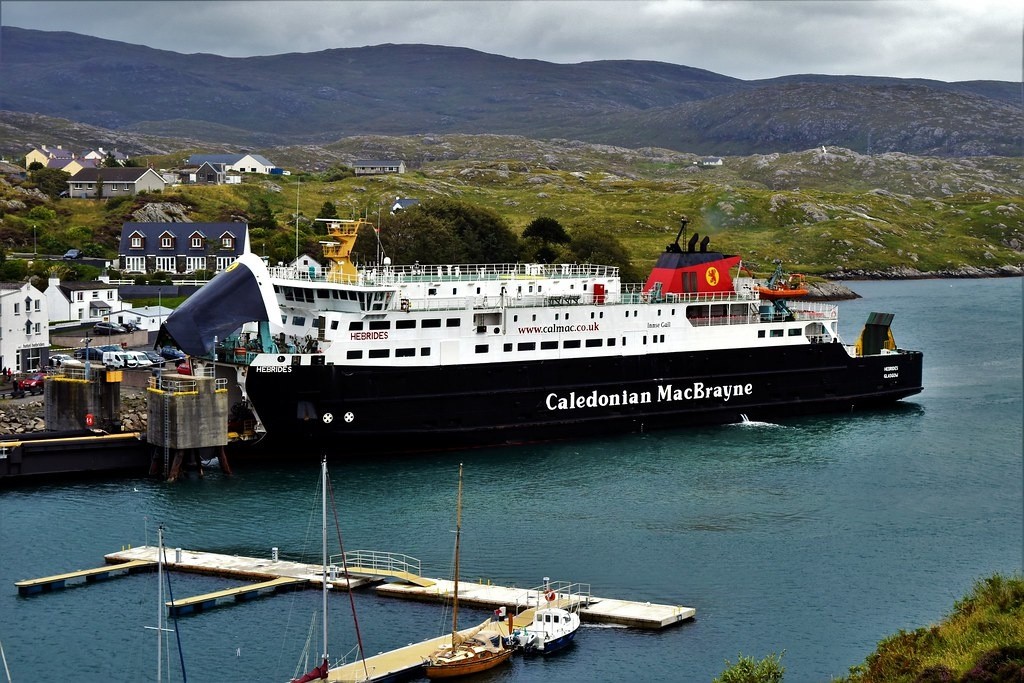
34 225 37 256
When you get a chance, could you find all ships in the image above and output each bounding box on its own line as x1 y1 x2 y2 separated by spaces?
158 180 927 462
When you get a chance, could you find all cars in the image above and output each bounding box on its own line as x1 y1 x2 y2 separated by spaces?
74 347 105 361
177 362 191 376
63 249 84 260
18 374 45 392
93 322 127 335
160 348 187 360
126 351 152 367
101 345 126 353
142 350 165 366
49 354 82 368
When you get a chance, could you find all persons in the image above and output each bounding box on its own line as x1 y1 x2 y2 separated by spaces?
2 359 61 391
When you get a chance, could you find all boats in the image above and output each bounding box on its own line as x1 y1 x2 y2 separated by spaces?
515 578 590 655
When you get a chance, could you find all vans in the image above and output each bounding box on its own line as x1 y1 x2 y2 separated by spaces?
102 351 139 369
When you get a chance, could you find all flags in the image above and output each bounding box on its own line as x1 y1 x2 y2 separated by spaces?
741 265 752 277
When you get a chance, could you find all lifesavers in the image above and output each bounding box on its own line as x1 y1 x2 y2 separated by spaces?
545 590 554 601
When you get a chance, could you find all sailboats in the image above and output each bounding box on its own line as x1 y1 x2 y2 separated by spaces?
418 462 516 679
